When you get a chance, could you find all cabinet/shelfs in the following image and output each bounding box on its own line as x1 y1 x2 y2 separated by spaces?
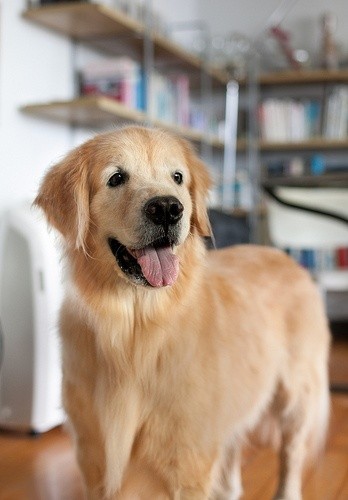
19 1 348 246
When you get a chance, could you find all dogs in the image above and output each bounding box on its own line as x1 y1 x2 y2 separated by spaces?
30 125 332 499
